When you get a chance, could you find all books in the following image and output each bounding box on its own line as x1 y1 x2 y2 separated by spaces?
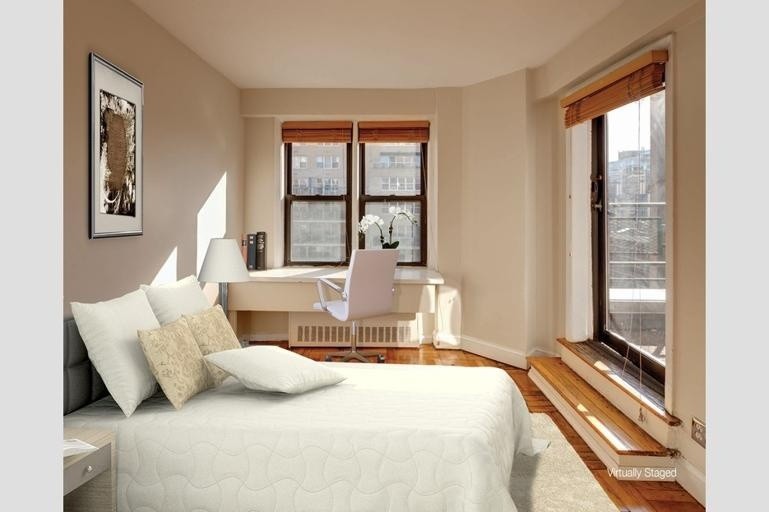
245 233 258 272
255 230 268 272
240 232 249 266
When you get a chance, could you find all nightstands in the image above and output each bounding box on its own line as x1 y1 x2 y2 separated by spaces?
64 427 118 511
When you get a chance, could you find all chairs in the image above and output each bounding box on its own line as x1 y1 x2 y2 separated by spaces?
315 249 399 363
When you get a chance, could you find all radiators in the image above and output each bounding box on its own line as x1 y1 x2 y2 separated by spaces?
289 312 423 350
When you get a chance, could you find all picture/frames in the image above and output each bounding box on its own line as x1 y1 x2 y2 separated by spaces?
87 51 145 238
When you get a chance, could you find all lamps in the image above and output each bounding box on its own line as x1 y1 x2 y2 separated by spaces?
199 239 252 312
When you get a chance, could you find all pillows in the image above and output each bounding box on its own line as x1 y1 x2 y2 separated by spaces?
184 303 243 385
141 274 210 323
71 282 166 417
136 315 211 409
204 345 346 394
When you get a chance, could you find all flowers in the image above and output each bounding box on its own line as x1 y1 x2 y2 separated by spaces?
355 209 416 241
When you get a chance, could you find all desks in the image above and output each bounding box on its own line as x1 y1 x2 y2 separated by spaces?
225 265 445 346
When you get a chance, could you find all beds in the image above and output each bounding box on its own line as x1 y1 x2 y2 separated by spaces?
64 311 545 512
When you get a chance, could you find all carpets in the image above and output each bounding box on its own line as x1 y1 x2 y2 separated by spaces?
526 411 620 512
